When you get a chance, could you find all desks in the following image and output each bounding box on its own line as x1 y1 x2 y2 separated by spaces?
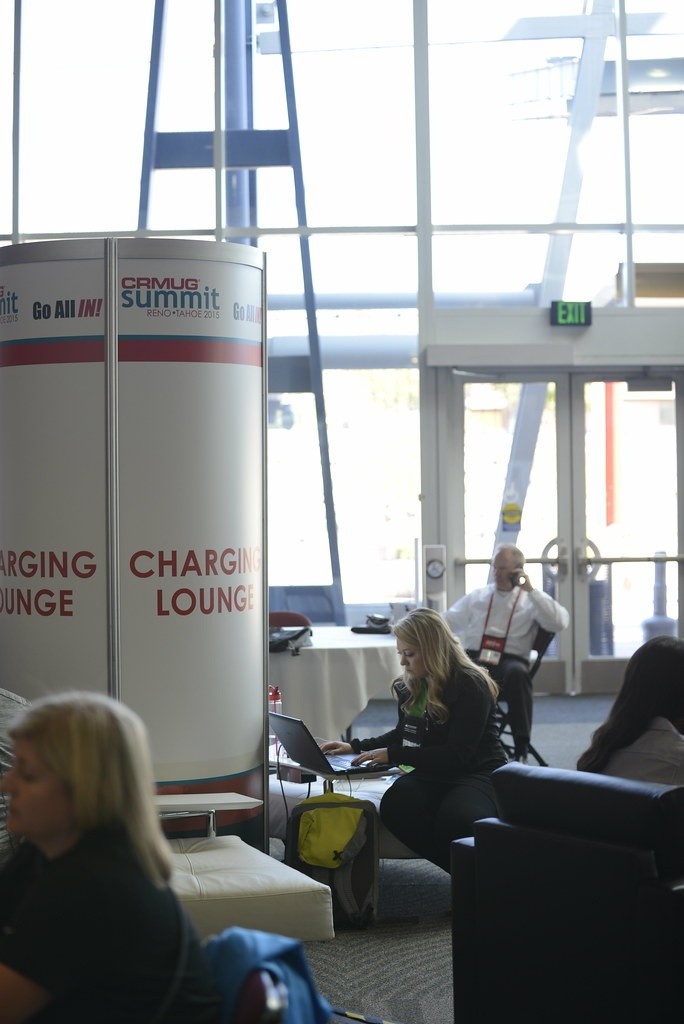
154 792 264 836
269 626 405 742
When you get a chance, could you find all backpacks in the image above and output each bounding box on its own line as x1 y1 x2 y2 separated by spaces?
283 795 379 929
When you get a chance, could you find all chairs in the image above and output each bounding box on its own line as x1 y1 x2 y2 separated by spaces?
269 611 311 626
489 626 555 768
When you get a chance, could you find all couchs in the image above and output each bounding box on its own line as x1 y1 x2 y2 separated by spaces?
450 762 684 1024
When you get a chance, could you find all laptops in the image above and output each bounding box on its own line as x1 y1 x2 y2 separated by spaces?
266 711 401 775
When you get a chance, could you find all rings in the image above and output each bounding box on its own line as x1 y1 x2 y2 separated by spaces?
372 755 375 759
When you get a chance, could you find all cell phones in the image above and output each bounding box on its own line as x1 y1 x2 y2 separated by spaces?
511 563 524 588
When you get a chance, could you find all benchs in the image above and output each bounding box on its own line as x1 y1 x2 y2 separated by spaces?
163 771 425 945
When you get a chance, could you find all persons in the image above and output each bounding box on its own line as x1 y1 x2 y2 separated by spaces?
0 691 201 1024
441 543 569 767
320 608 508 875
577 635 684 786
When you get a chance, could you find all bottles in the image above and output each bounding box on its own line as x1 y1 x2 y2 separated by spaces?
267 684 284 763
